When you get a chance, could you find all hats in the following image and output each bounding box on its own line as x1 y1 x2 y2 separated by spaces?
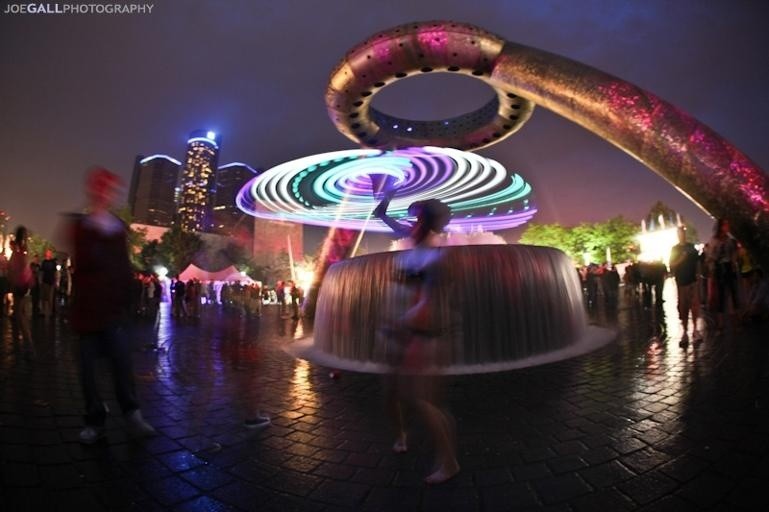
409 200 450 230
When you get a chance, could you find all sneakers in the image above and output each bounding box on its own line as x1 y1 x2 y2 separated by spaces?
246 415 271 428
129 409 156 436
81 403 111 442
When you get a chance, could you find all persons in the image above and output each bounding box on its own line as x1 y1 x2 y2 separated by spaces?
0 221 304 360
704 215 745 338
669 226 704 346
576 237 756 309
370 198 463 485
55 162 156 447
